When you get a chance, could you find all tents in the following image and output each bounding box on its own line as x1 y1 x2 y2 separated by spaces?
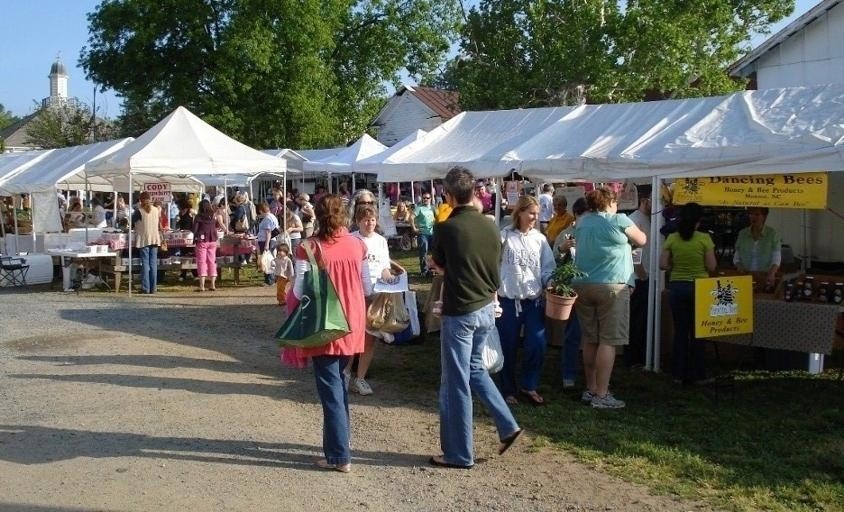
83 105 287 296
302 132 387 192
205 143 312 236
0 134 136 257
350 128 431 204
375 80 844 373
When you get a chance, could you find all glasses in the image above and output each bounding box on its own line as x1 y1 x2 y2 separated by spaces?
422 196 431 201
357 201 375 206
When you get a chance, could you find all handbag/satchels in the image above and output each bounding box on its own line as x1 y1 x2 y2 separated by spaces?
482 314 503 377
256 251 275 274
273 239 352 350
378 309 427 347
157 237 168 252
365 274 411 336
382 289 421 348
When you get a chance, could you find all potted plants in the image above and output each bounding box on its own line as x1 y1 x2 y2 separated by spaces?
545 263 590 322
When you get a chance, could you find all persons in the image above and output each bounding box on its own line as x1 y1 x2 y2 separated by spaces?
170 194 179 227
117 197 130 222
288 195 367 472
313 186 326 202
434 178 444 203
733 206 782 290
344 189 393 395
271 244 295 307
270 189 284 216
388 202 410 250
543 195 575 244
104 192 114 226
234 189 257 287
214 198 230 236
553 198 591 389
154 200 170 231
428 165 524 470
414 183 426 201
295 192 316 238
539 183 556 232
571 186 647 410
0 146 58 256
486 177 497 194
258 204 280 285
282 201 304 239
133 192 161 293
175 199 200 282
410 193 435 278
231 190 252 264
474 178 492 211
193 200 227 291
89 198 107 224
339 185 351 195
436 192 453 222
501 195 558 407
659 203 717 387
555 182 566 189
63 202 88 229
625 187 651 368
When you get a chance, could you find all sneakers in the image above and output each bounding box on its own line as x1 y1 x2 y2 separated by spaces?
563 374 577 394
209 287 216 291
693 377 717 388
351 378 375 396
137 291 150 294
580 390 618 403
343 372 352 392
591 394 626 410
150 291 157 294
672 379 684 388
316 459 351 473
276 302 288 307
194 288 206 293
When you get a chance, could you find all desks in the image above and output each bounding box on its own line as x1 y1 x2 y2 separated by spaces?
96 235 197 295
707 289 841 373
69 227 102 243
4 252 53 284
217 232 259 286
47 248 117 289
5 230 69 255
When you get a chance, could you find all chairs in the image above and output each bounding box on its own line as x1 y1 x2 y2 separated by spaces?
0 252 30 290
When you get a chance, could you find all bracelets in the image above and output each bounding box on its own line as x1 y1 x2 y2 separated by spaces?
770 267 779 279
556 246 566 257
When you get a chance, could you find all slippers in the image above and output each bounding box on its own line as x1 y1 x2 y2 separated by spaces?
523 390 546 407
497 427 524 456
428 455 475 470
506 397 517 409
